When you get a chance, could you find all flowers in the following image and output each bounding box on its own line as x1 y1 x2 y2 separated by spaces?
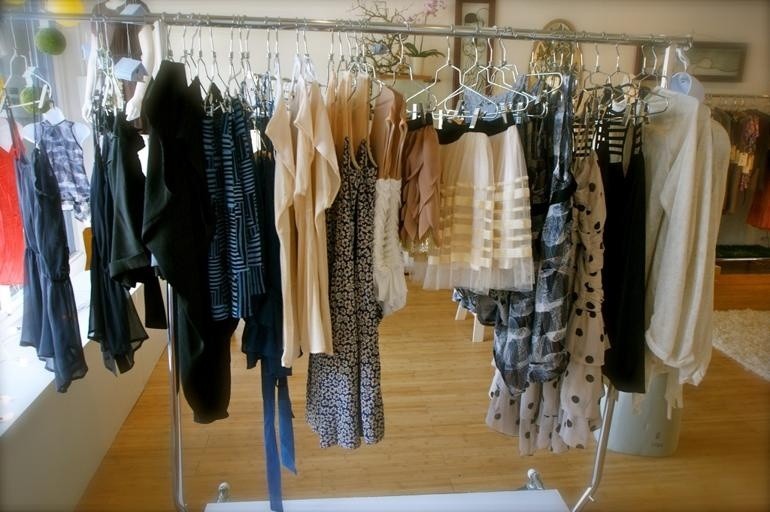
395 0 446 59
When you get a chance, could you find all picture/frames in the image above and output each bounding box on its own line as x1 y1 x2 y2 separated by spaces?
451 0 497 109
633 40 749 82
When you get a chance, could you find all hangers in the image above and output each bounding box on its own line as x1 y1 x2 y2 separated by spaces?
701 93 770 115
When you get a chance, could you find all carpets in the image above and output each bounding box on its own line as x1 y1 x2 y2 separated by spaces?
710 309 770 387
